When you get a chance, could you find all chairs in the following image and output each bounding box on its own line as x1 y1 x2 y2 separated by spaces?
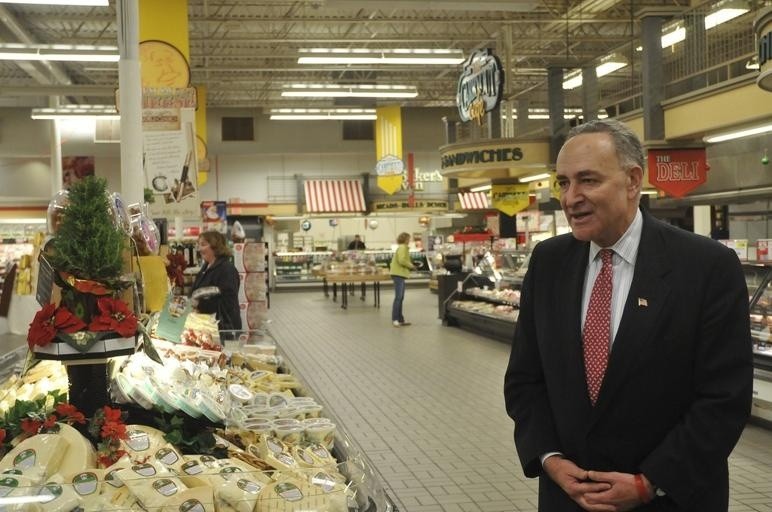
393 319 411 327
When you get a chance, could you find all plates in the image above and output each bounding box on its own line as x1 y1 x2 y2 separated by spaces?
436 244 528 339
0 293 398 510
740 257 771 426
272 250 431 291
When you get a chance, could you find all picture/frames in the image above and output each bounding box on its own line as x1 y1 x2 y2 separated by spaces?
313 267 394 311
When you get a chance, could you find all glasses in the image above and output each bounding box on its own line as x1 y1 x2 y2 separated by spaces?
266 44 467 126
0 40 125 127
501 0 754 124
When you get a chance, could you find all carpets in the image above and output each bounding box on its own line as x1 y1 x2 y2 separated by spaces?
581 249 615 412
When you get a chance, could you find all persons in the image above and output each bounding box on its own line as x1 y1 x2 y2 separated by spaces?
502 118 756 512
225 218 247 243
347 234 368 251
389 230 417 327
474 254 483 265
186 230 243 339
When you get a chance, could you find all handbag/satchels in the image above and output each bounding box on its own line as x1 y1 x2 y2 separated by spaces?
631 472 649 507
